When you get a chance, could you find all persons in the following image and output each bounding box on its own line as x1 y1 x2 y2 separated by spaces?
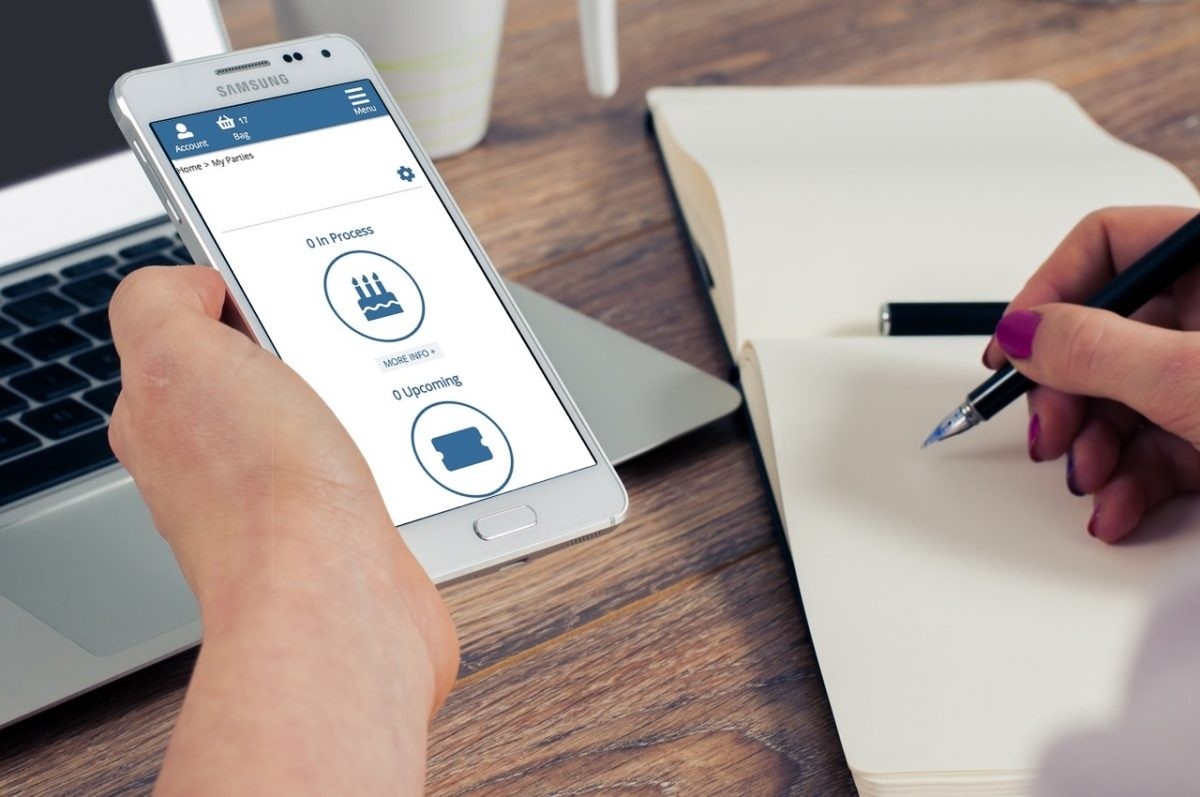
105 206 1200 797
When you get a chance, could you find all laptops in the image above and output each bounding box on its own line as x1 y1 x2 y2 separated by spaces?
1 0 744 730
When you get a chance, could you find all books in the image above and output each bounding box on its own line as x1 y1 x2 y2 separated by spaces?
645 80 1200 797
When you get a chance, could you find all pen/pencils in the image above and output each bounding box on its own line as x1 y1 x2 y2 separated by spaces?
929 215 1200 445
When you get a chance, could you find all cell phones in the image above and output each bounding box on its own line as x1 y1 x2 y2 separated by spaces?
107 31 630 589
